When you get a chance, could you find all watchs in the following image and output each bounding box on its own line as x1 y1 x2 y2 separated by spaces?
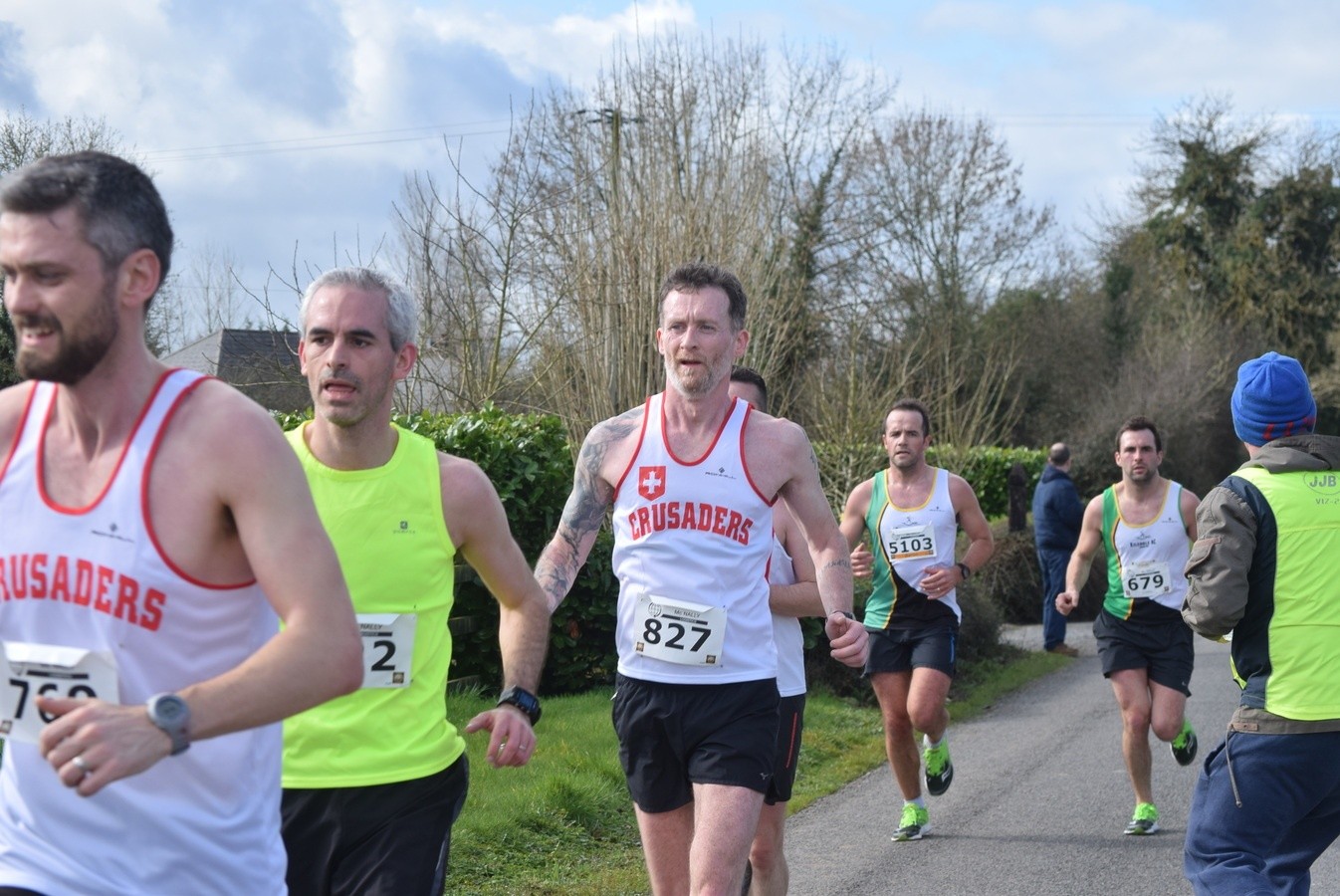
832 610 856 622
146 691 193 756
956 562 971 579
496 685 542 725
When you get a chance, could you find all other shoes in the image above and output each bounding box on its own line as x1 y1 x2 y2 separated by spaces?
1048 644 1077 656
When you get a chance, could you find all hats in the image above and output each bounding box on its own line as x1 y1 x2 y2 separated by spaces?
1231 351 1316 447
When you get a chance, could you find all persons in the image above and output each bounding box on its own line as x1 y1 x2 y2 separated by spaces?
0 153 362 896
535 266 869 896
726 368 826 896
1032 442 1087 657
1055 416 1201 834
837 398 995 843
1180 350 1340 896
278 267 549 896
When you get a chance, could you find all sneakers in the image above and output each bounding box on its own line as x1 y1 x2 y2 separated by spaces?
891 803 931 842
1169 720 1197 767
1124 803 1159 835
923 733 953 795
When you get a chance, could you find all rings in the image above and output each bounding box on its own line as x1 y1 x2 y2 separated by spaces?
72 755 87 772
